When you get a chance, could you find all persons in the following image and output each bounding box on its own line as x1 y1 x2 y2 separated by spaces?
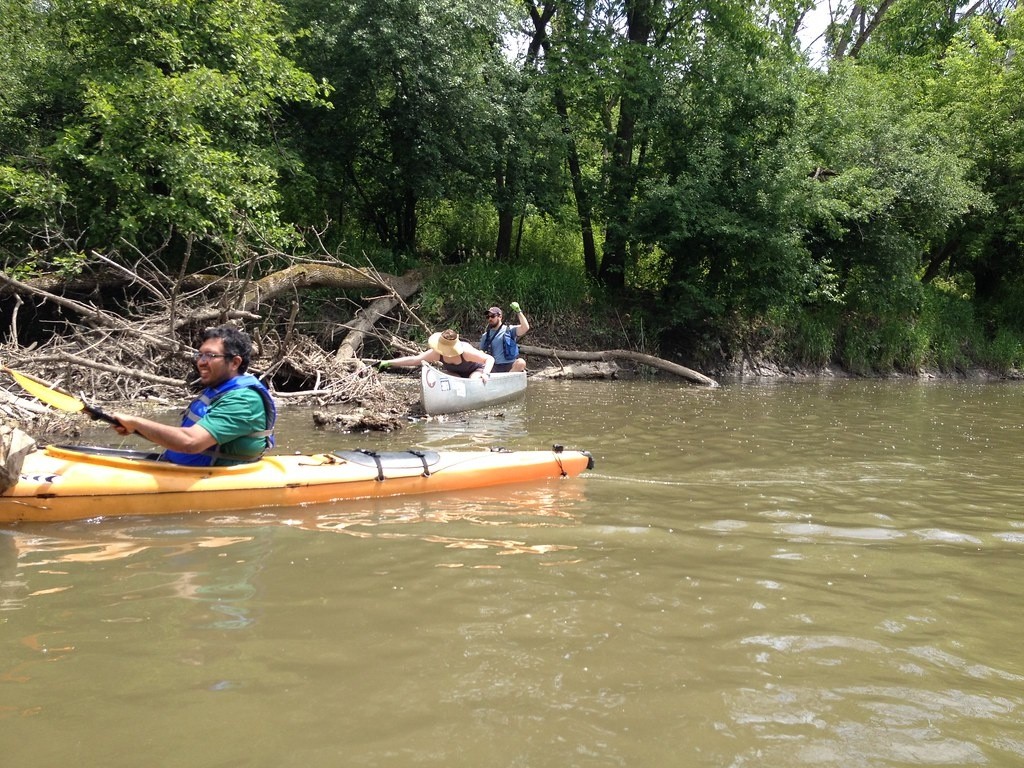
112 325 276 467
480 302 530 373
375 330 494 383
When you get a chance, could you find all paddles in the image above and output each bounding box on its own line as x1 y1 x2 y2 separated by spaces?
11 368 149 440
483 309 513 352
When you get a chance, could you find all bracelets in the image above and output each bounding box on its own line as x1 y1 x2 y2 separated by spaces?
483 371 491 377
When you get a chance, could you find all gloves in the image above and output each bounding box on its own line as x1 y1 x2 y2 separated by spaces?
373 360 390 373
509 302 522 313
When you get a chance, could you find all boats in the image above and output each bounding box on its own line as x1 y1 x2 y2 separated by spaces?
1 443 595 527
420 360 528 416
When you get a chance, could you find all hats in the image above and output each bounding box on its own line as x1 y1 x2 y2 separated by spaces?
427 331 464 357
484 307 502 315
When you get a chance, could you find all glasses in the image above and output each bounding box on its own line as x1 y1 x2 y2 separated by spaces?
486 314 497 319
194 352 240 360
